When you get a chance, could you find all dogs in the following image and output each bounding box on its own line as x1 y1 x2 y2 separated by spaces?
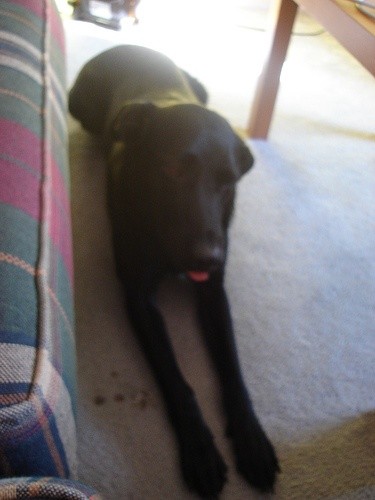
67 42 285 499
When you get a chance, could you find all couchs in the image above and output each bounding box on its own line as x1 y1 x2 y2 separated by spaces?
0 0 375 499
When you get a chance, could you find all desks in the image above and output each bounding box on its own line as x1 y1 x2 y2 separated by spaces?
247 0 375 148
